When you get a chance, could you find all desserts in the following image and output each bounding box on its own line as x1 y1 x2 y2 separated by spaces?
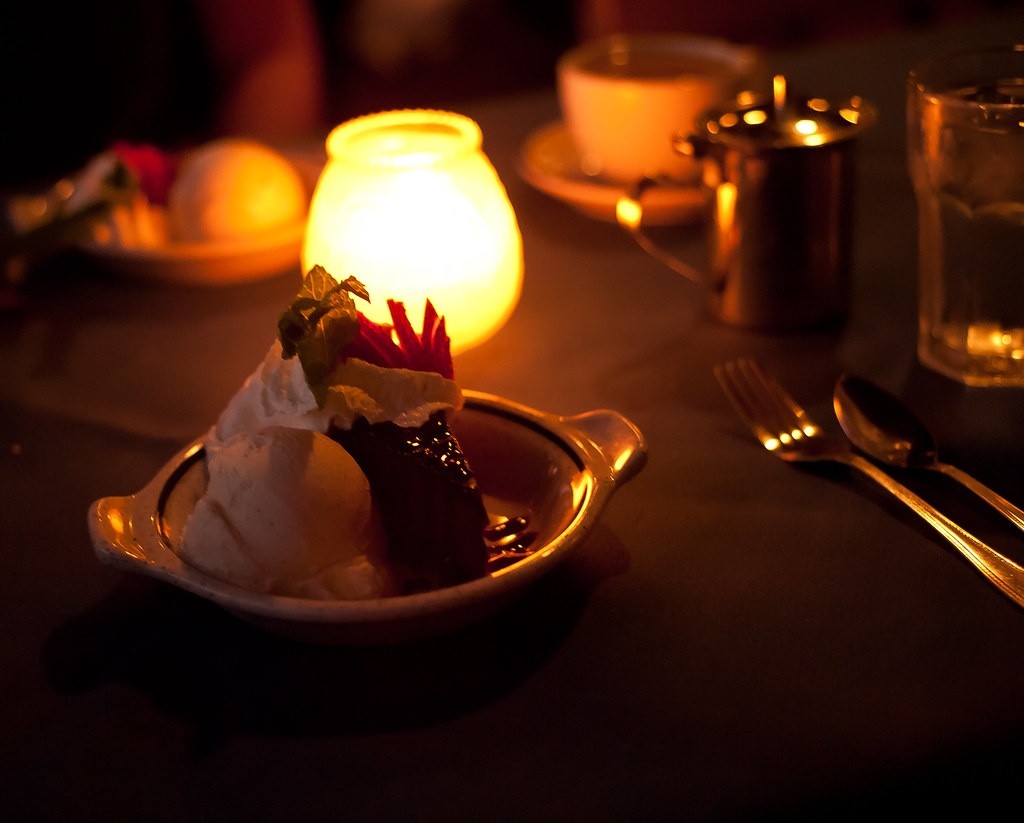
176 265 498 602
81 133 305 261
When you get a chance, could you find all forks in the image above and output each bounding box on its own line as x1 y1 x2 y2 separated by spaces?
716 355 1024 610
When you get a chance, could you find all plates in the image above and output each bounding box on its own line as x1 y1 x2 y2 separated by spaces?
88 389 643 643
517 124 713 226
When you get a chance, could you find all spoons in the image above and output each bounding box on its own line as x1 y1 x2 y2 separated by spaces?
833 374 1024 533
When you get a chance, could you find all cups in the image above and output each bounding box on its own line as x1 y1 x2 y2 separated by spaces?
907 44 1024 387
628 104 874 333
558 39 758 170
